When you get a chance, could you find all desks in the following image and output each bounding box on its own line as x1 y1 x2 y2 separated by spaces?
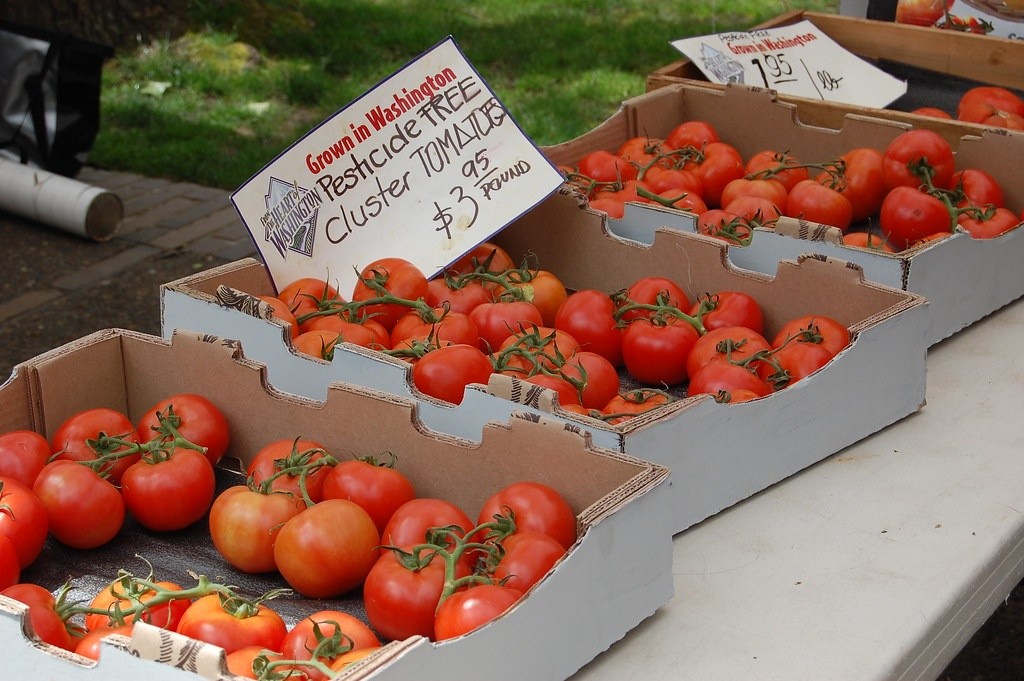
578 293 1024 681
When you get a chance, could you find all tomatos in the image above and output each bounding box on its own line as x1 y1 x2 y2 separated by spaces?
0 389 574 681
551 119 1024 254
909 86 1024 131
893 0 995 37
251 241 849 425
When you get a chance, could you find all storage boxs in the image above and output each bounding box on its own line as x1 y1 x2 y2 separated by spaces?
641 6 1024 151
0 322 679 681
151 192 933 540
838 0 1024 41
524 79 1024 355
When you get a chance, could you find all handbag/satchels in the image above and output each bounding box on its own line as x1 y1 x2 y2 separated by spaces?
0 25 115 176
